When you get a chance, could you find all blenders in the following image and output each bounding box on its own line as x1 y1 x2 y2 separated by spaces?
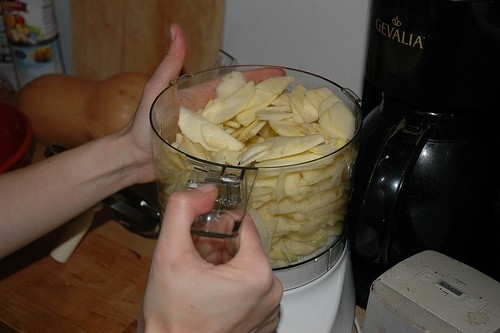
146 63 361 333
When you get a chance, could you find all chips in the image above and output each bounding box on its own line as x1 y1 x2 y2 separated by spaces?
153 70 358 267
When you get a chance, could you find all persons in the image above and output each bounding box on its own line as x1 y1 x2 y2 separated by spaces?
0 23 287 333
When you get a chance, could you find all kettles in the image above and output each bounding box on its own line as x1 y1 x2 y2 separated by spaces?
340 74 486 315
